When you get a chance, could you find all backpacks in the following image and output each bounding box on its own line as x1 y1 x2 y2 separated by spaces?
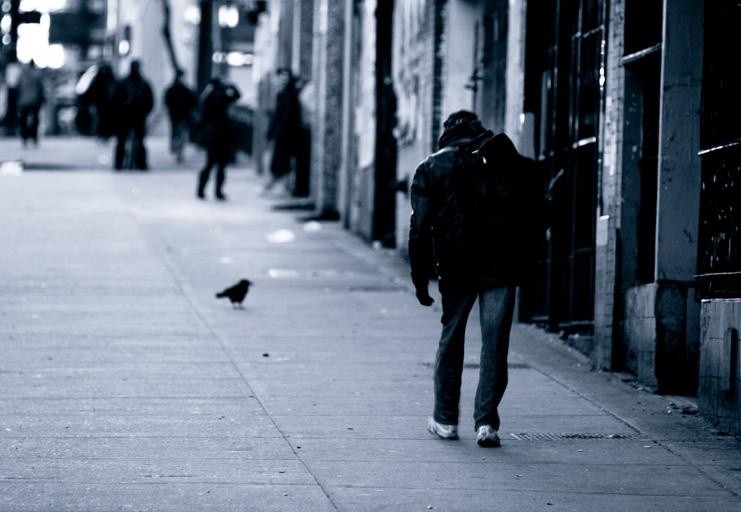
448 133 551 241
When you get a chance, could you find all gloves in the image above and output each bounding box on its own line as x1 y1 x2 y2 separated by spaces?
411 266 438 306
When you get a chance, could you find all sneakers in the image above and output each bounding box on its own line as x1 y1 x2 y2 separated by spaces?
427 417 457 440
476 424 501 446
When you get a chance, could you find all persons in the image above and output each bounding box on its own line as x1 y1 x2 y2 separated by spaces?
16 59 47 147
90 60 153 170
404 111 542 447
264 68 302 193
164 70 195 160
198 79 240 198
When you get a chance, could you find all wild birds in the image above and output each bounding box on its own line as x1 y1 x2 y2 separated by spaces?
216 279 252 310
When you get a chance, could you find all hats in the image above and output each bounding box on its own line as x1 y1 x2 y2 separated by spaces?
438 110 487 150
